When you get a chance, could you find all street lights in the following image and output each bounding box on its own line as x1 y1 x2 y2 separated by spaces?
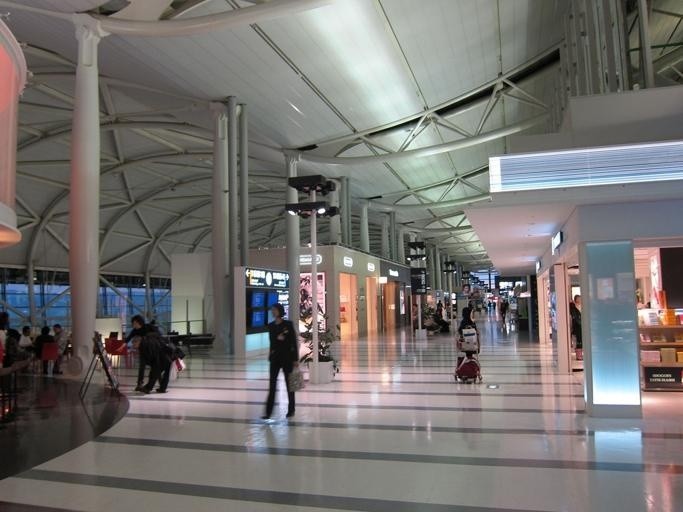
407 241 428 339
282 173 340 386
442 259 457 328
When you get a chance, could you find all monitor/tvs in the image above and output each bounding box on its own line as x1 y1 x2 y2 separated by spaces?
250 290 267 309
266 308 277 326
265 292 279 308
250 309 266 328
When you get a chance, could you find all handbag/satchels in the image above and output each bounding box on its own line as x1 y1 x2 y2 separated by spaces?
167 343 185 381
289 367 303 392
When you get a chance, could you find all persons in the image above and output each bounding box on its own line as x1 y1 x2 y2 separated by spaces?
0 312 66 376
458 307 480 358
260 303 298 419
569 295 582 360
468 300 509 318
433 300 449 333
115 315 173 394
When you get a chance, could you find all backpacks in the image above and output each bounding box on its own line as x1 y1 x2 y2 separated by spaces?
461 325 478 351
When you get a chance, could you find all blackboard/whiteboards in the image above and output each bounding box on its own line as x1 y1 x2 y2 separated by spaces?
92 333 119 388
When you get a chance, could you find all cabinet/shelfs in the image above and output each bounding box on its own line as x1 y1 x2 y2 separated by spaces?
637 308 683 392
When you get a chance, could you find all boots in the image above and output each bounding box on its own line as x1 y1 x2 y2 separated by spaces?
575 348 582 360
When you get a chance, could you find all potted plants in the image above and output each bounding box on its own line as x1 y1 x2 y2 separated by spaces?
297 284 341 383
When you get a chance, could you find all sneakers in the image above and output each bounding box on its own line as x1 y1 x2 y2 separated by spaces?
135 384 166 393
